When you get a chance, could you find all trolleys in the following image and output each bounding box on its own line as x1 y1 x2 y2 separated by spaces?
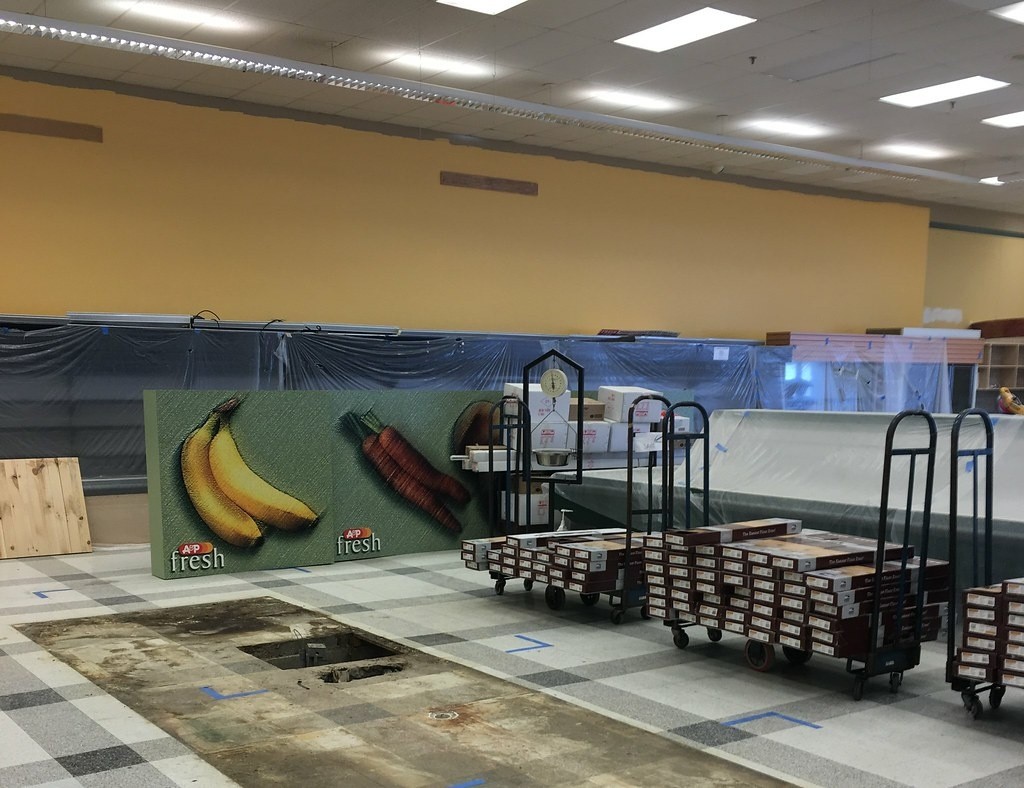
661 400 937 703
486 395 675 628
944 407 1024 721
